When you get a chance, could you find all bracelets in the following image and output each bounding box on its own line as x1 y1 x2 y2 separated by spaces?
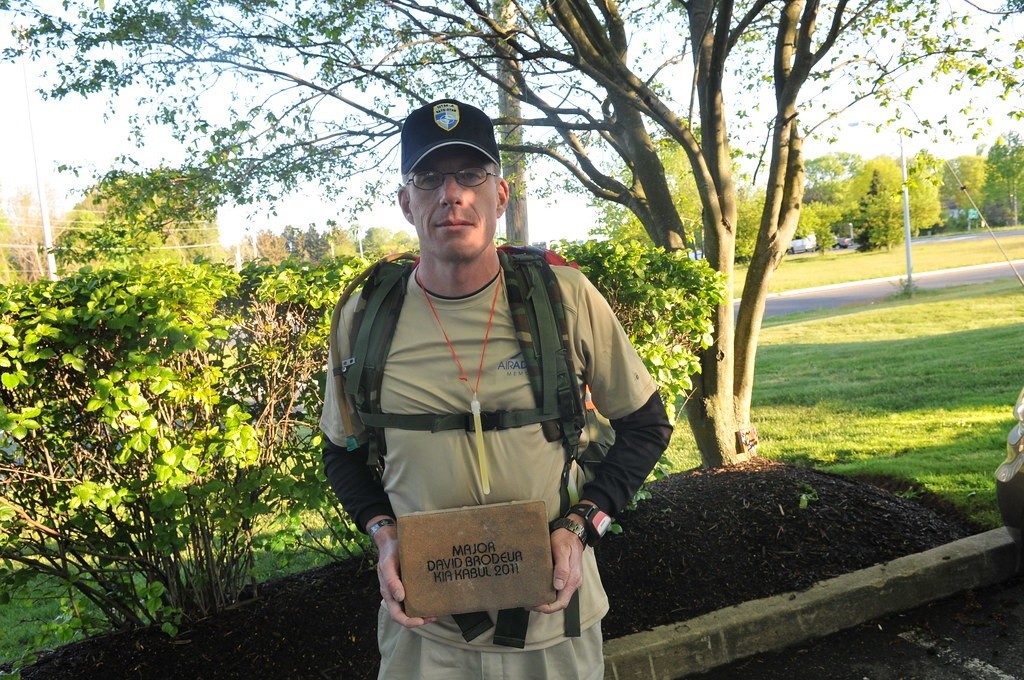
549 517 587 550
568 504 613 546
368 518 398 543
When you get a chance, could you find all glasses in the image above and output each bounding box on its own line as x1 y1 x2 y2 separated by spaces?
406 167 498 190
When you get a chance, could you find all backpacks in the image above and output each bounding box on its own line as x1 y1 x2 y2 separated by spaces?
330 245 616 648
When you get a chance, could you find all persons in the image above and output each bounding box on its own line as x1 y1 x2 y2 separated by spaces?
313 99 676 680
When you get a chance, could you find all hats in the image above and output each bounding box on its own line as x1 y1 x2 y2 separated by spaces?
401 99 501 178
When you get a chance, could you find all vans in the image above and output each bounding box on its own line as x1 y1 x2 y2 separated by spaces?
786 234 818 255
